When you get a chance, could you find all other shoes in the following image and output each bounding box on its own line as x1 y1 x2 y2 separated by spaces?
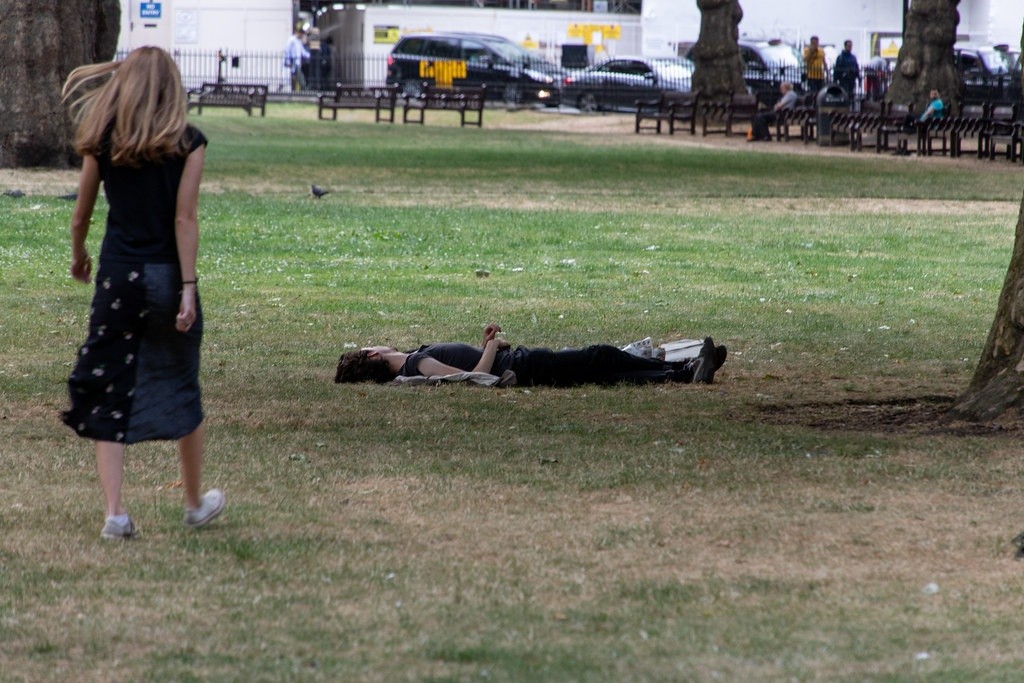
763 135 772 141
748 134 760 142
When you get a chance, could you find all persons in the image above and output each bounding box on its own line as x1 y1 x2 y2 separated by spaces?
803 36 829 94
746 82 798 141
832 40 862 113
334 322 727 390
284 27 338 91
59 43 226 542
895 87 945 134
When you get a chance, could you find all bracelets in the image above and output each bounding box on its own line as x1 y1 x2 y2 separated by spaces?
183 278 198 284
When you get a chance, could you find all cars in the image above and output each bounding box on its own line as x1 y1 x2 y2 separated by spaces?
953 47 1023 105
561 53 754 118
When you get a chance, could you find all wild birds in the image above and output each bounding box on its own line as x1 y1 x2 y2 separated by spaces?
311 184 330 200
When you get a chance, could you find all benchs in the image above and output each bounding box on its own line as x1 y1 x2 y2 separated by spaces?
318 82 399 121
401 81 489 127
187 81 268 116
633 90 1024 164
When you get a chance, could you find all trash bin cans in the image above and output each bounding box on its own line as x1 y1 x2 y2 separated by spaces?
816 85 849 147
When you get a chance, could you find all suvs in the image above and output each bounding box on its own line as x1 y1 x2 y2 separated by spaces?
683 38 809 108
385 31 572 111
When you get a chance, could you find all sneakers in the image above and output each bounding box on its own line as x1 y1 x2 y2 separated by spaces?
714 345 727 372
182 489 226 528
683 335 714 384
100 517 140 541
